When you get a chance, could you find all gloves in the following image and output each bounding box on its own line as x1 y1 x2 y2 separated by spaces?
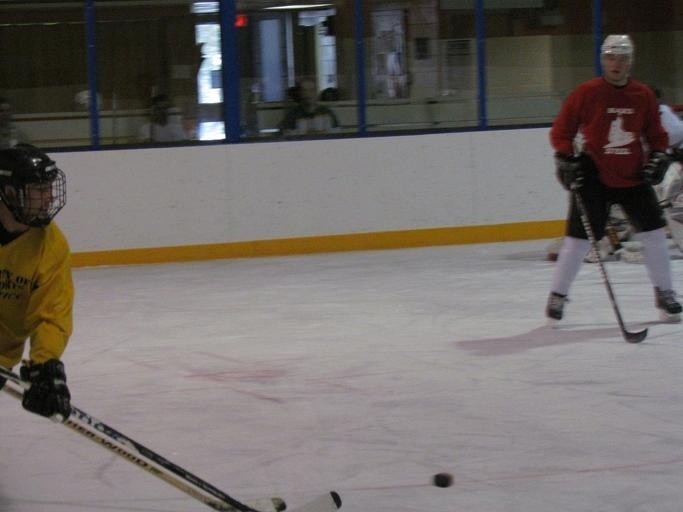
551 151 583 190
642 149 673 185
18 356 72 420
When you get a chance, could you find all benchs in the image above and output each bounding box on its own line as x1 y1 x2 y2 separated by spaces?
2 71 561 148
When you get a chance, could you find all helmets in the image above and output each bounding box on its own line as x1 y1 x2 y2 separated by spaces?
0 142 59 218
600 34 634 73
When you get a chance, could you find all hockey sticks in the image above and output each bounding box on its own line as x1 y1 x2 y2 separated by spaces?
2 367 288 511
1 374 341 511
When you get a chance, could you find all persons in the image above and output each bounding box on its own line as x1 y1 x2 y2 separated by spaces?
284 79 338 139
136 95 200 143
0 97 31 145
545 32 683 321
0 142 76 424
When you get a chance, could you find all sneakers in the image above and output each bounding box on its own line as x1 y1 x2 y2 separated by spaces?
653 284 683 317
546 291 569 322
